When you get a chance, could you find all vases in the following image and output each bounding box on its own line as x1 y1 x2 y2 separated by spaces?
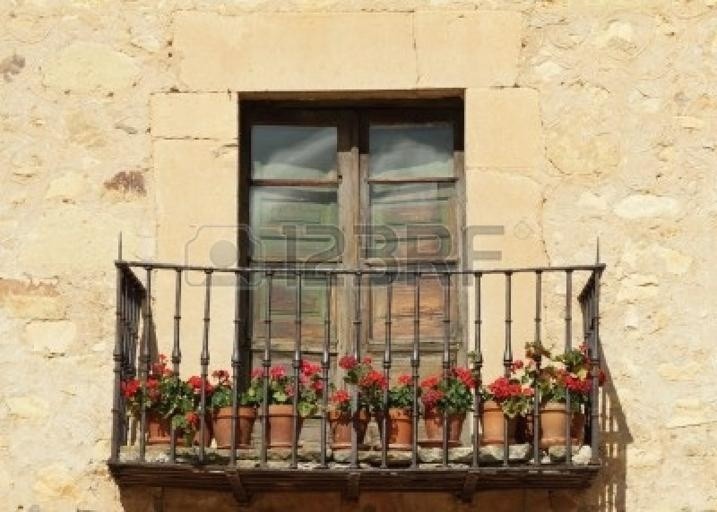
145 403 577 446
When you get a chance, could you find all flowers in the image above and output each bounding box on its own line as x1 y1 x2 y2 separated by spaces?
118 337 604 427
